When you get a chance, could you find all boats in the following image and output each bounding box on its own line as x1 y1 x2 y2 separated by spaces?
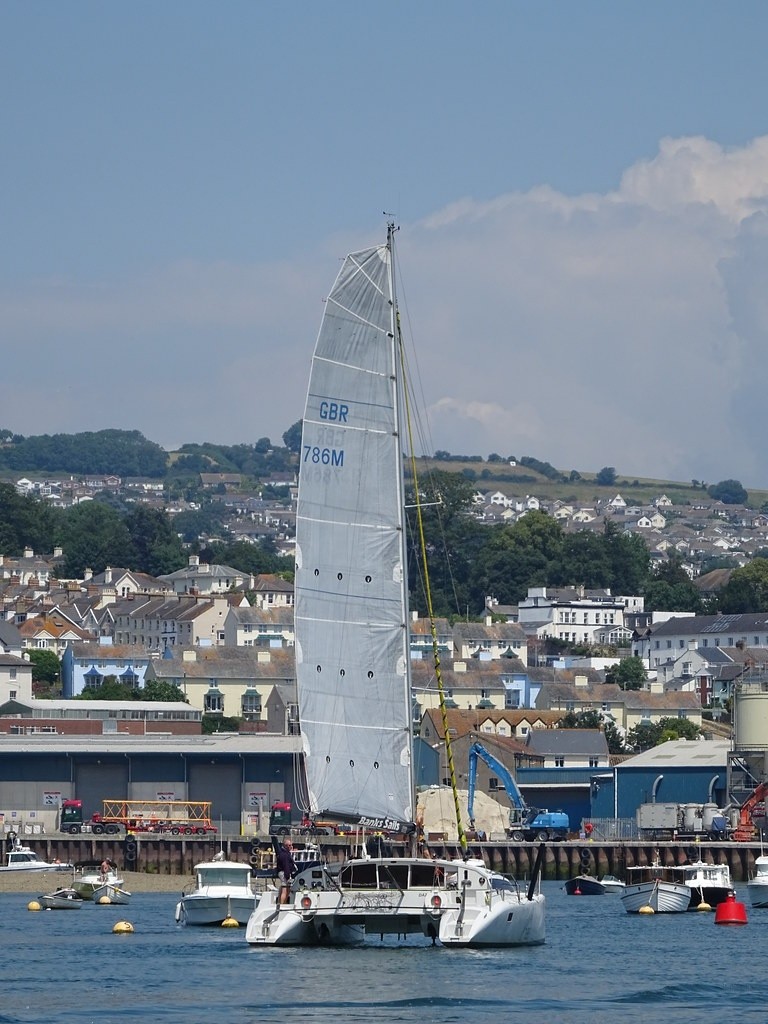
625 858 736 902
70 858 123 900
601 874 623 894
91 883 133 905
746 853 768 910
621 880 692 916
174 854 263 926
0 837 75 874
564 874 606 896
37 887 83 909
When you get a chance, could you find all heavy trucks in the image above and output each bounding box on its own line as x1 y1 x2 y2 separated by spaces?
267 801 407 842
59 798 219 837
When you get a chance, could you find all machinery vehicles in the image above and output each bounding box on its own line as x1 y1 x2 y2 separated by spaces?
707 780 768 840
456 739 571 842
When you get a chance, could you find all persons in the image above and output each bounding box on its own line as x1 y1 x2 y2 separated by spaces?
366 831 391 861
276 837 299 904
101 858 112 881
56 859 61 864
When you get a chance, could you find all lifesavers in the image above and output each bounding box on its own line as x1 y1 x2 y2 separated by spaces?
295 890 318 916
425 890 449 914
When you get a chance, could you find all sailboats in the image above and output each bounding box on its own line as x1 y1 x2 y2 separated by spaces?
242 208 550 957
